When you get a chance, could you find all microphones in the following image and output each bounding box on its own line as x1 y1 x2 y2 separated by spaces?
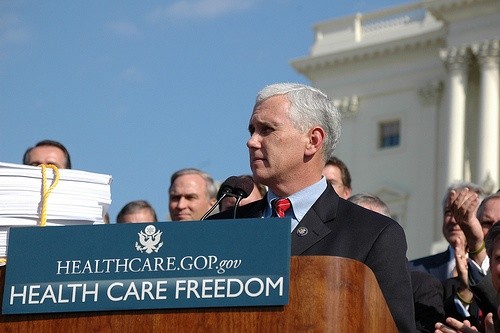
200 176 254 221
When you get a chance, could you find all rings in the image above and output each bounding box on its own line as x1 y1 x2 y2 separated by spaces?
458 254 462 259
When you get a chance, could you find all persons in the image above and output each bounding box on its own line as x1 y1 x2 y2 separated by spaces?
347 193 391 217
408 180 500 333
116 200 157 224
23 140 71 169
217 175 267 213
169 168 219 222
321 156 352 200
206 83 415 333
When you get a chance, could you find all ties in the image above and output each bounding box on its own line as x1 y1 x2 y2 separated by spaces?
271 197 291 218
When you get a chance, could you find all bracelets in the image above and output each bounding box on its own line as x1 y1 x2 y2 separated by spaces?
455 291 473 304
465 240 486 255
